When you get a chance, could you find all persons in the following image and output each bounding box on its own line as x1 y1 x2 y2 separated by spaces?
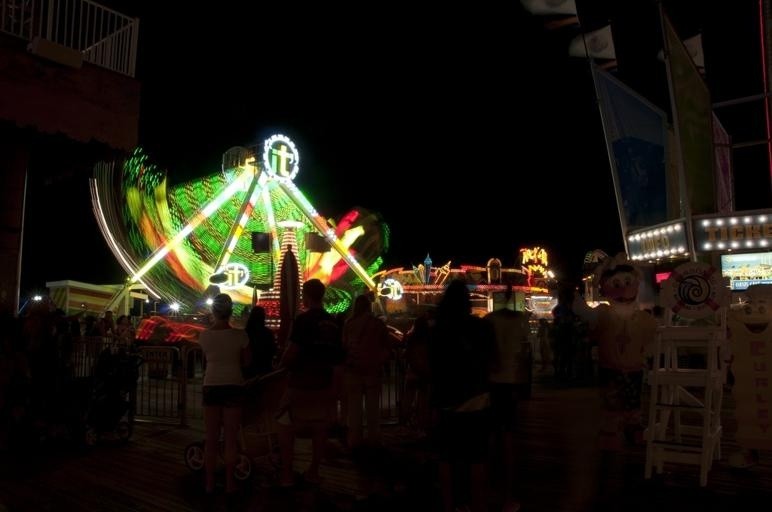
536 318 550 373
93 311 135 338
572 252 658 456
198 278 531 495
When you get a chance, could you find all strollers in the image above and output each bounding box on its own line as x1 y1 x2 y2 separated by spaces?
184 359 294 484
47 352 140 447
